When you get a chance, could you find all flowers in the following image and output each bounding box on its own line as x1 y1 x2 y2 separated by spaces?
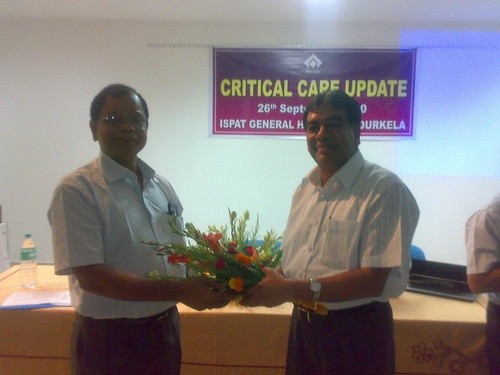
136 204 330 315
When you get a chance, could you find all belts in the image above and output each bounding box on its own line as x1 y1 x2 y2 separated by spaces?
296 304 333 325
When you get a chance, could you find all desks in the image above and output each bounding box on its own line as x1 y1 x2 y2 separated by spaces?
0 261 491 375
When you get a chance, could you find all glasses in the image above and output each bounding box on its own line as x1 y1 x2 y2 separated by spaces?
95 113 147 128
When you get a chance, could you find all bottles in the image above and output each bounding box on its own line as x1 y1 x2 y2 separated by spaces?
20 233 37 288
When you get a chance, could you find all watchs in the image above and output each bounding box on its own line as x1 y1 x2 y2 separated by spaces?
309 277 322 303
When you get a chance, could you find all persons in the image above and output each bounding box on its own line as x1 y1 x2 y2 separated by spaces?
464 201 500 375
239 90 421 375
47 83 235 374
